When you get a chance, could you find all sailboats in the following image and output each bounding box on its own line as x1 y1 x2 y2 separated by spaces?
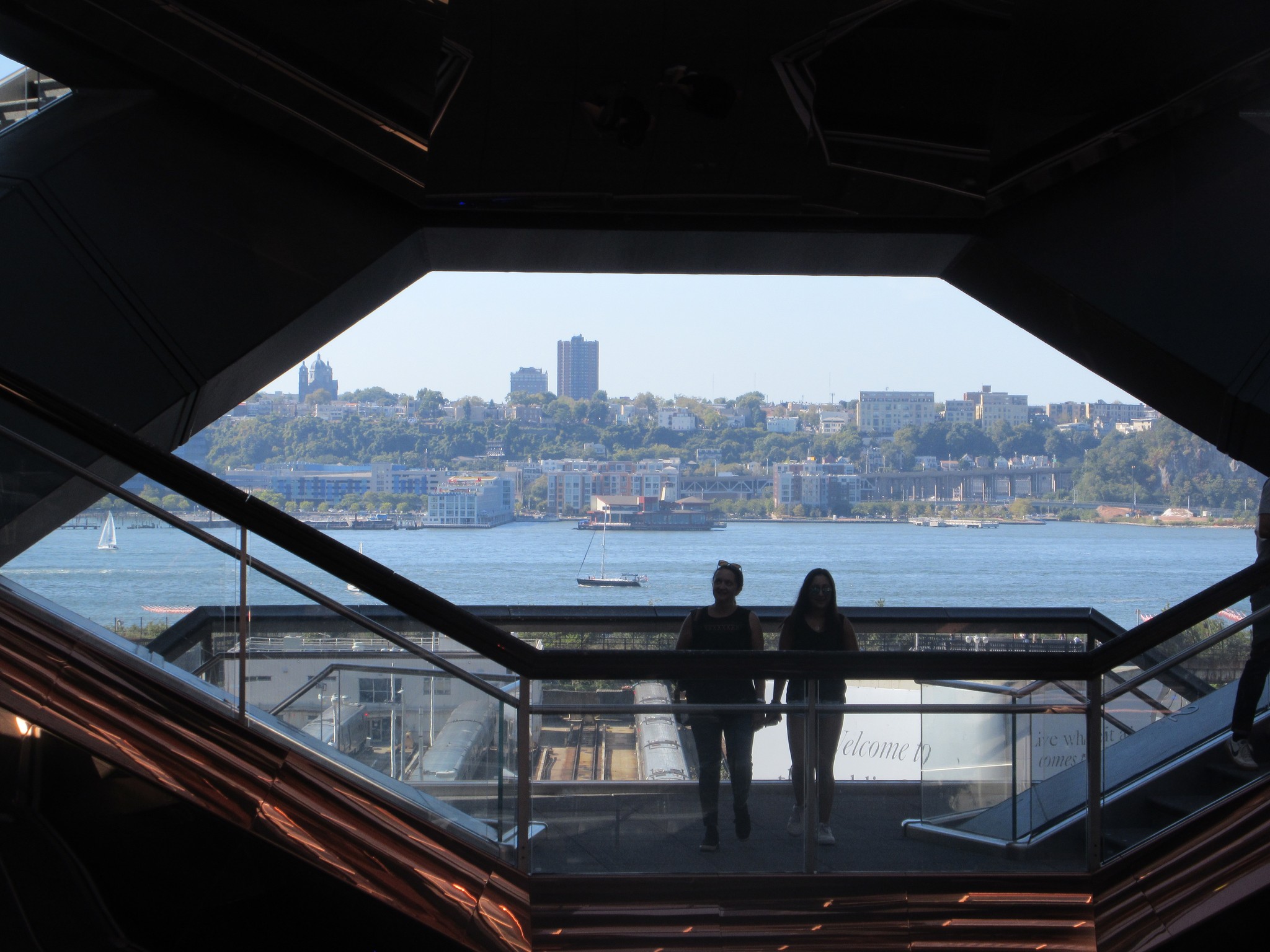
98 510 120 550
576 502 640 587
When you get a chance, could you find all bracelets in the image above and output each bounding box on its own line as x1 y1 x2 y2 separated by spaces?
757 698 766 703
771 700 781 704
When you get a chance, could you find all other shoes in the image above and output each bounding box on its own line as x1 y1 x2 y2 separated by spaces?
733 804 751 841
818 824 835 845
701 828 719 852
787 805 805 837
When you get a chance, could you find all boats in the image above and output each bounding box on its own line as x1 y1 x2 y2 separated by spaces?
630 510 712 531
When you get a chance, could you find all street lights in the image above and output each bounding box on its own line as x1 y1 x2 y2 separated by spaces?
1014 451 1018 469
949 454 952 471
1084 449 1088 465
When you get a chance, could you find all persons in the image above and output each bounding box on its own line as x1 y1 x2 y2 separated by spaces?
767 568 860 844
673 561 768 851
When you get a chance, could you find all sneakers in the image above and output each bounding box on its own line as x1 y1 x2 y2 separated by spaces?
1224 736 1258 769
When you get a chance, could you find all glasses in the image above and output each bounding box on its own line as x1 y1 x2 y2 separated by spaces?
717 560 743 575
812 586 832 594
